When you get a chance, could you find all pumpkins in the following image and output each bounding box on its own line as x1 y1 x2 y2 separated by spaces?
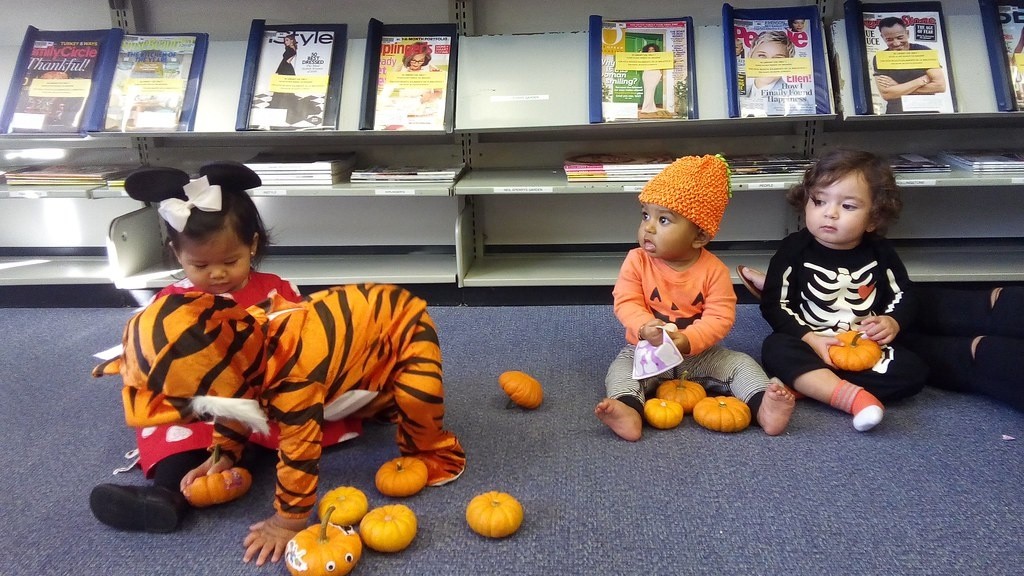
284 487 417 576
465 490 524 538
374 456 428 497
643 370 751 432
498 371 543 410
828 330 882 371
182 443 253 507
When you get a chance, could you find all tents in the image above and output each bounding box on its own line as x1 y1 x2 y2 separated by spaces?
374 36 451 130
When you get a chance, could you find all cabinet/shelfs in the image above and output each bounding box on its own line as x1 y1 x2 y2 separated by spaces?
0 0 1024 310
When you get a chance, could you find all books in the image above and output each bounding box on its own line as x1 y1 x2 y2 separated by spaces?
6 152 468 186
249 31 334 130
9 34 197 136
565 149 1024 182
732 18 831 116
601 21 688 121
863 12 954 114
1000 5 1024 108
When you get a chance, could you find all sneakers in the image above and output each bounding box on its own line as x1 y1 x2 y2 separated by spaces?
90 483 187 533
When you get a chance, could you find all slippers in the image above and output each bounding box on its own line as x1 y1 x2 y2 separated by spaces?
736 264 765 300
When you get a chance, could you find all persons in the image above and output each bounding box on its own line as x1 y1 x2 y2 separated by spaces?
91 163 268 530
594 156 797 441
641 44 663 113
760 149 927 430
735 19 814 115
737 265 1024 411
567 155 672 164
267 35 322 125
91 283 465 565
390 41 443 115
873 17 946 114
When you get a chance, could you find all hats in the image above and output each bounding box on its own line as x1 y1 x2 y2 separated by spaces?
637 153 732 239
